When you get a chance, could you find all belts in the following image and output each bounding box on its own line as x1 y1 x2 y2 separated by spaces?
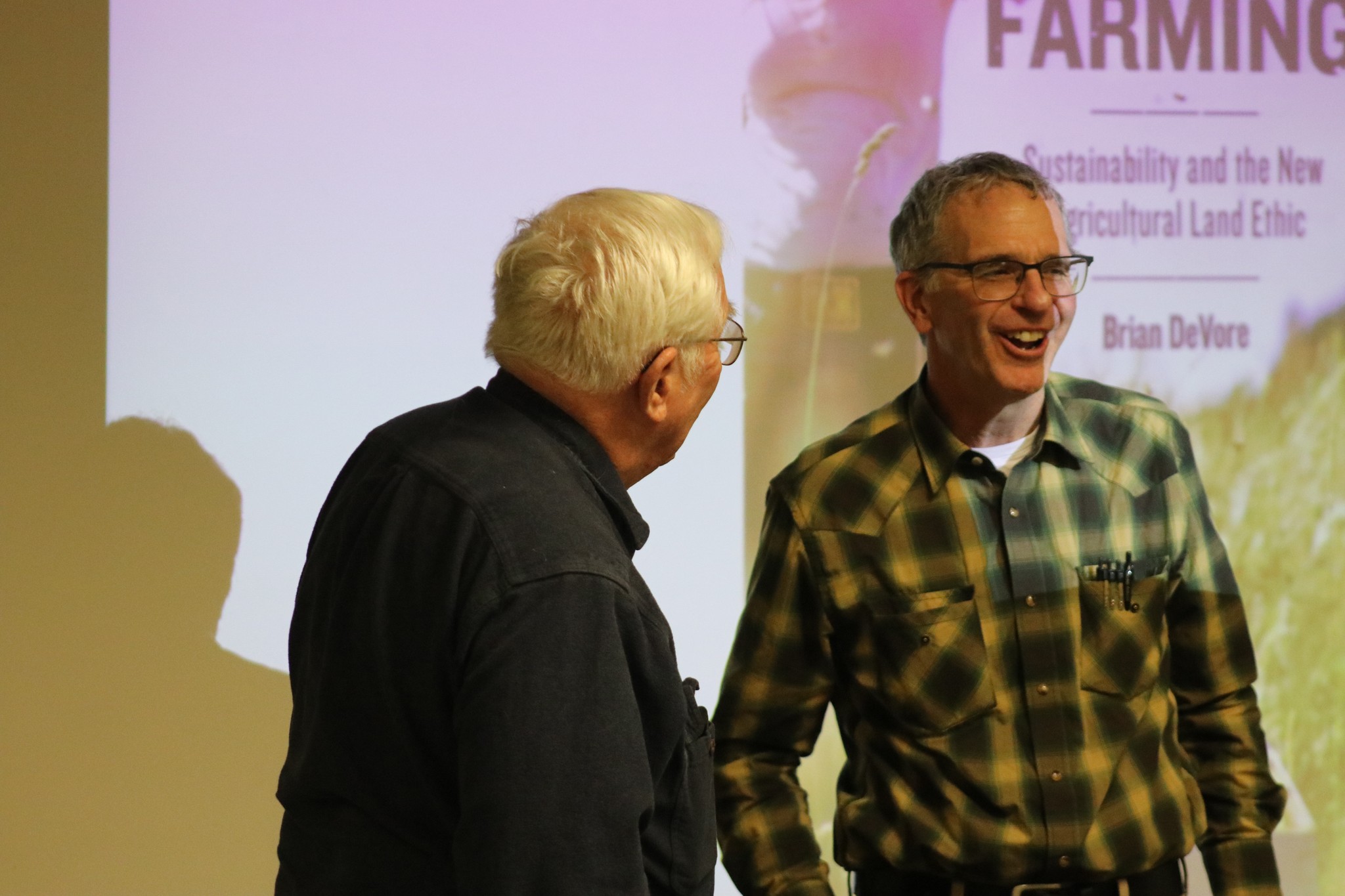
854 854 1186 896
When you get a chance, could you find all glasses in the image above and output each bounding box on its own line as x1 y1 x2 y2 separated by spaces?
641 314 748 365
915 253 1094 302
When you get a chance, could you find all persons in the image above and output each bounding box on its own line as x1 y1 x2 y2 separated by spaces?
268 187 747 895
710 150 1298 894
